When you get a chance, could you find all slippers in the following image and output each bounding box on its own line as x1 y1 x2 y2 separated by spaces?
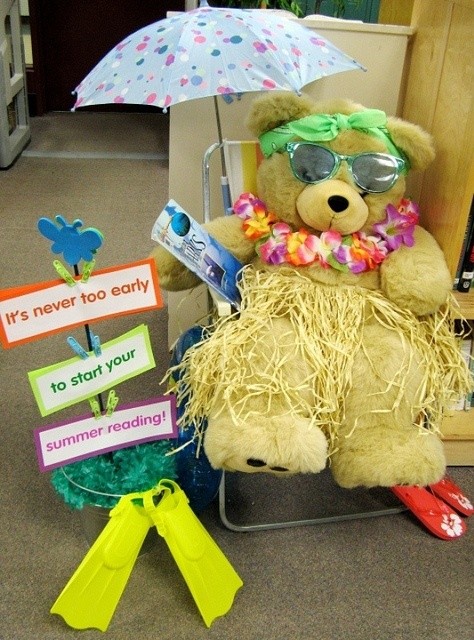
430 479 473 516
392 485 467 541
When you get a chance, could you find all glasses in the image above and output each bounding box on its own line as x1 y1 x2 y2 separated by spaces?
280 139 408 192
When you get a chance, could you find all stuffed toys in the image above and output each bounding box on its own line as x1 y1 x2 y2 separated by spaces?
147 91 474 490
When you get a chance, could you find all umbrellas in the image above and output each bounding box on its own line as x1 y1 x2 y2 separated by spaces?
71 0 367 217
